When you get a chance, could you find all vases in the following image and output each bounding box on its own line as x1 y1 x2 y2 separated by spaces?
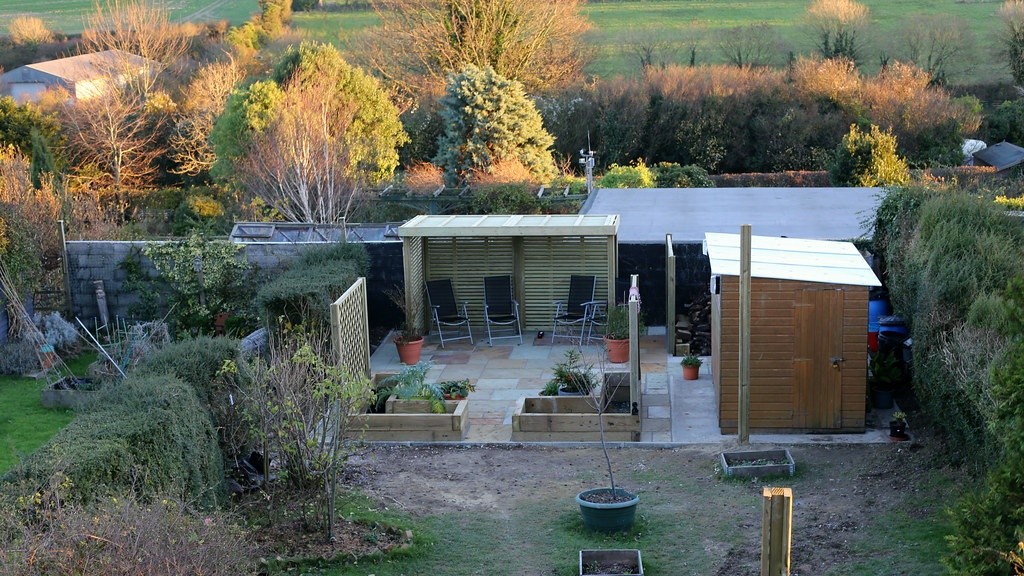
576 486 639 531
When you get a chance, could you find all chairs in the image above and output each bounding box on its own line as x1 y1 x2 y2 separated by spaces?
552 275 607 347
483 275 523 347
425 278 473 349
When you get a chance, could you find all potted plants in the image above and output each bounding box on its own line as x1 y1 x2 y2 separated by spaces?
867 351 902 410
721 449 795 477
369 360 476 414
579 548 644 576
603 303 645 363
889 412 908 436
538 348 600 397
680 353 703 380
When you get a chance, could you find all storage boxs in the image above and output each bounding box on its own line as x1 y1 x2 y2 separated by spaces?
676 343 689 356
